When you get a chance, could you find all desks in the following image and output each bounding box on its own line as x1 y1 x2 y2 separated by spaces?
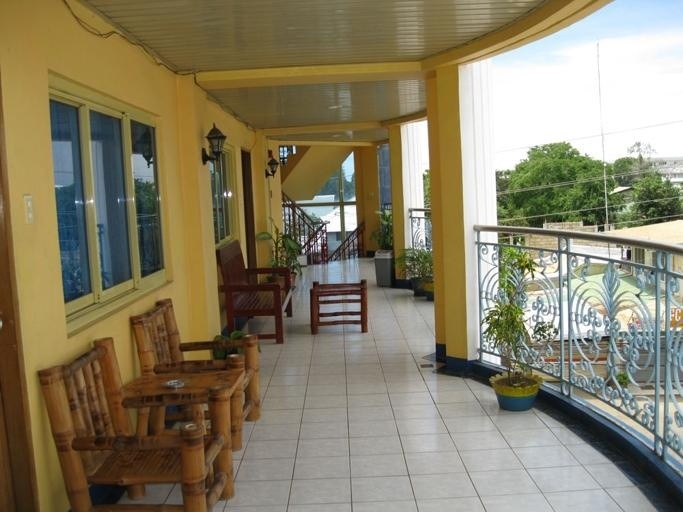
116 362 249 489
305 276 372 336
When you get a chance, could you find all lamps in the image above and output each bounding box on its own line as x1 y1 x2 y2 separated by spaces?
200 120 228 166
263 155 279 181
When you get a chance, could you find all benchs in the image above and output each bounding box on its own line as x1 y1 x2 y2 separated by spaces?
213 237 298 345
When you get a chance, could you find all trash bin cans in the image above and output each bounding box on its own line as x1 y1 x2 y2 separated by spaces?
375 250 394 287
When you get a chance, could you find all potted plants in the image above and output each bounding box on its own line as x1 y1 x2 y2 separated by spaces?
254 214 302 289
472 245 562 416
390 244 427 300
414 248 437 303
278 238 304 291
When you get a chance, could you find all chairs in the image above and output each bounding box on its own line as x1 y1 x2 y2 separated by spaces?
127 295 269 455
36 336 240 512
